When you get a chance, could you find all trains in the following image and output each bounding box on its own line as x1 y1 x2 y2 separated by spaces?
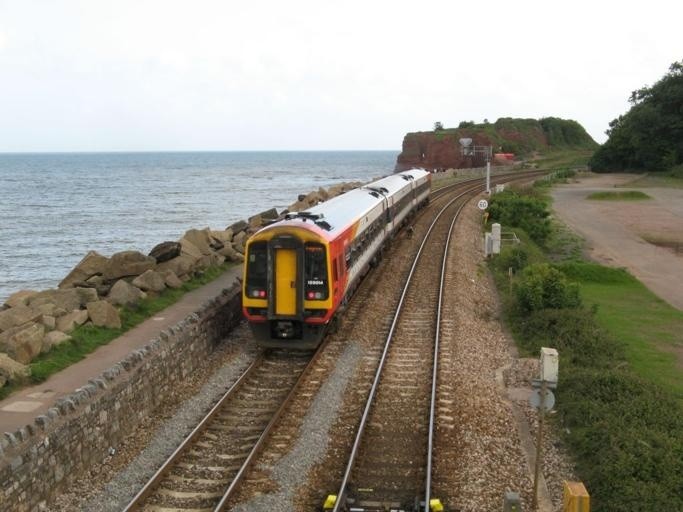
238 163 434 360
